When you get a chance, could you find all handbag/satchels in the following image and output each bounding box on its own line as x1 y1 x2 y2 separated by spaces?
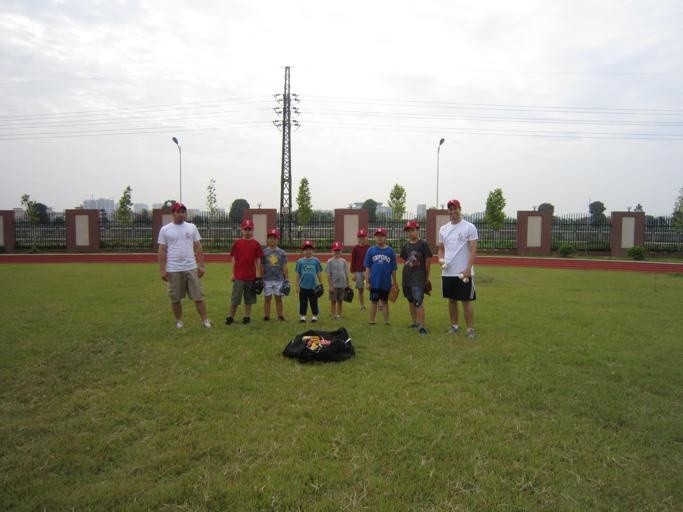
282 327 355 363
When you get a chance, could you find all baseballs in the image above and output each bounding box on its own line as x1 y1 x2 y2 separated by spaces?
463 277 467 282
441 265 445 269
439 258 444 264
457 274 463 278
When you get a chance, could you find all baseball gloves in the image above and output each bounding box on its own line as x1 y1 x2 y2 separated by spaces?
389 283 399 303
343 287 353 302
278 280 291 295
424 280 432 295
313 284 323 297
252 279 263 294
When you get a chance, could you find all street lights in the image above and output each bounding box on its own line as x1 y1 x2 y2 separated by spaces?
436 138 444 209
171 136 183 205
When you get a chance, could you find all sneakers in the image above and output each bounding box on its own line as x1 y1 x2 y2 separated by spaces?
175 302 475 338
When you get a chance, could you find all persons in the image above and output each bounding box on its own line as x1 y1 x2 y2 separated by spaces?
224 219 266 325
261 228 290 322
324 241 352 321
363 228 399 325
349 229 374 313
400 221 434 335
156 202 215 329
294 240 325 324
437 199 479 340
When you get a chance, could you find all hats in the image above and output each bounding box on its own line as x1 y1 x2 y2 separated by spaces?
171 202 187 211
238 199 461 251
